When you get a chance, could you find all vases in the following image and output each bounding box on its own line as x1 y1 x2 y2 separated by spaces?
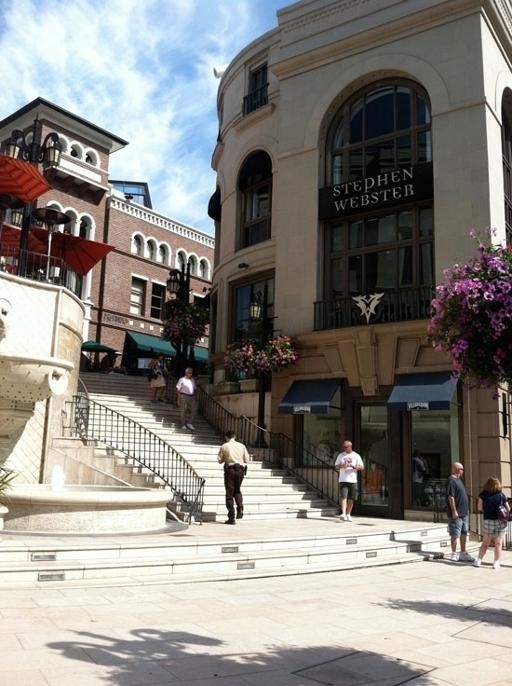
238 378 263 392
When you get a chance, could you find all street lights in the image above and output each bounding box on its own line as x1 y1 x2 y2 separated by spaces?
165 261 197 380
2 111 66 280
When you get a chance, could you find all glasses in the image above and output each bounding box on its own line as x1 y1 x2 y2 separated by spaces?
342 446 350 449
458 468 464 470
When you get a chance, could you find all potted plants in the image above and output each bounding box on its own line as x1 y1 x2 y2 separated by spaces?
221 372 240 393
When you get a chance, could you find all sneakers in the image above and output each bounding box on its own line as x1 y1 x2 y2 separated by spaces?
181 425 184 429
346 514 353 522
450 550 501 568
340 514 348 521
187 423 195 430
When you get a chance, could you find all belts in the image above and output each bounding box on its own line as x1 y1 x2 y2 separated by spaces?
228 466 243 469
180 392 195 397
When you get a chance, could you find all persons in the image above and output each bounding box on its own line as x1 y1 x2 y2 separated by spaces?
148 351 169 404
334 440 364 522
412 448 428 506
175 367 198 430
470 475 511 569
217 430 250 525
444 461 476 563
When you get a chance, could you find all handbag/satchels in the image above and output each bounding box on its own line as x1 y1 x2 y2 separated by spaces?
498 505 512 522
147 368 156 382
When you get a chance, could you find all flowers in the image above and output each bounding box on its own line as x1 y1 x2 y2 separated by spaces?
426 224 510 392
223 335 304 380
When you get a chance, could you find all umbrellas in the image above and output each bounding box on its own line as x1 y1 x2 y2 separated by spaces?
0 153 53 216
0 221 32 259
24 223 118 278
82 339 119 364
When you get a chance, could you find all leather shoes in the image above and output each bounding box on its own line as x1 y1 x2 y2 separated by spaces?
225 519 236 524
236 510 243 518
